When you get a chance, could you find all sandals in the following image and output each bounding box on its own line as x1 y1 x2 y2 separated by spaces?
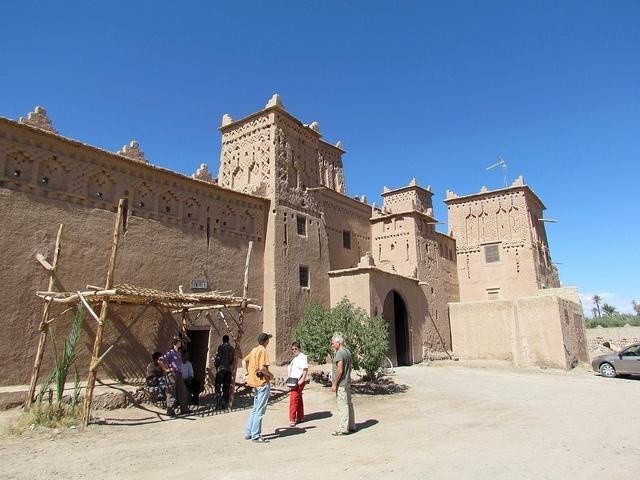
245 437 251 440
349 430 356 433
253 438 269 443
333 430 347 436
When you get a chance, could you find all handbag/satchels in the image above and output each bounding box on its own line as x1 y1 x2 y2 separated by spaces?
286 377 298 388
255 364 269 378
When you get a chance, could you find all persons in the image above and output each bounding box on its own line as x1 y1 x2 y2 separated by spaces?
287 342 309 427
241 333 274 443
214 335 235 410
181 349 199 405
158 336 193 416
331 335 356 436
145 352 166 401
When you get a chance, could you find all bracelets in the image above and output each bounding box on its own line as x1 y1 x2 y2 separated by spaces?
245 374 248 376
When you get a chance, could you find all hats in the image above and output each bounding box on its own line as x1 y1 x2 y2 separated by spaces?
257 333 272 344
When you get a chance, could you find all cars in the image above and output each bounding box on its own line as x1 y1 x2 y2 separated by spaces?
591 343 639 384
